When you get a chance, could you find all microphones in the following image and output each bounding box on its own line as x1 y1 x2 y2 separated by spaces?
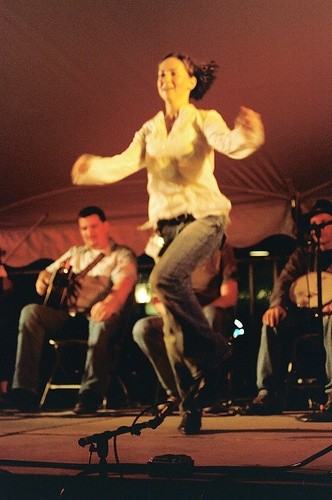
291 193 305 239
152 393 182 430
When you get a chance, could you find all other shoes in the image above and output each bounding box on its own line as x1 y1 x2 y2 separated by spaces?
0 388 38 410
177 411 202 434
152 393 182 411
245 393 278 413
73 396 103 412
322 395 332 415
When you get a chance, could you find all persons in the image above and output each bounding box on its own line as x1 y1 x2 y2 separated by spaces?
71 51 266 435
241 200 332 418
0 207 138 414
132 233 238 414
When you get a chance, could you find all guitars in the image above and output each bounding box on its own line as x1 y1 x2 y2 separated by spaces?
288 270 332 310
42 252 105 309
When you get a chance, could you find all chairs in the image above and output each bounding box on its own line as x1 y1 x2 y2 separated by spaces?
38 339 130 412
